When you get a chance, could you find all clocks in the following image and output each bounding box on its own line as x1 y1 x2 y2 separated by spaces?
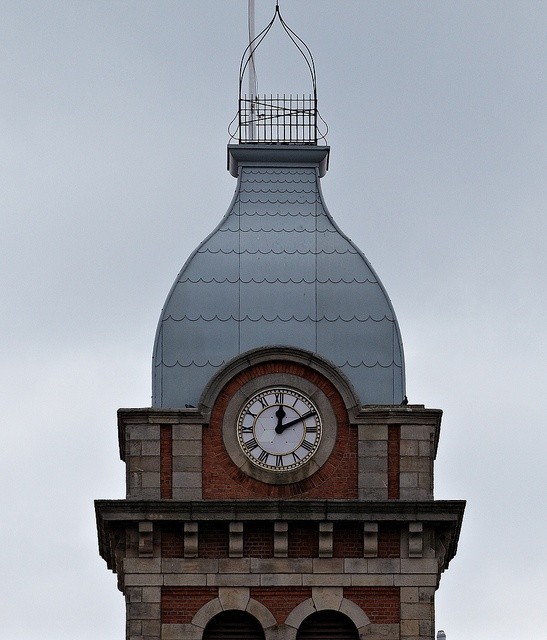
221 372 338 487
